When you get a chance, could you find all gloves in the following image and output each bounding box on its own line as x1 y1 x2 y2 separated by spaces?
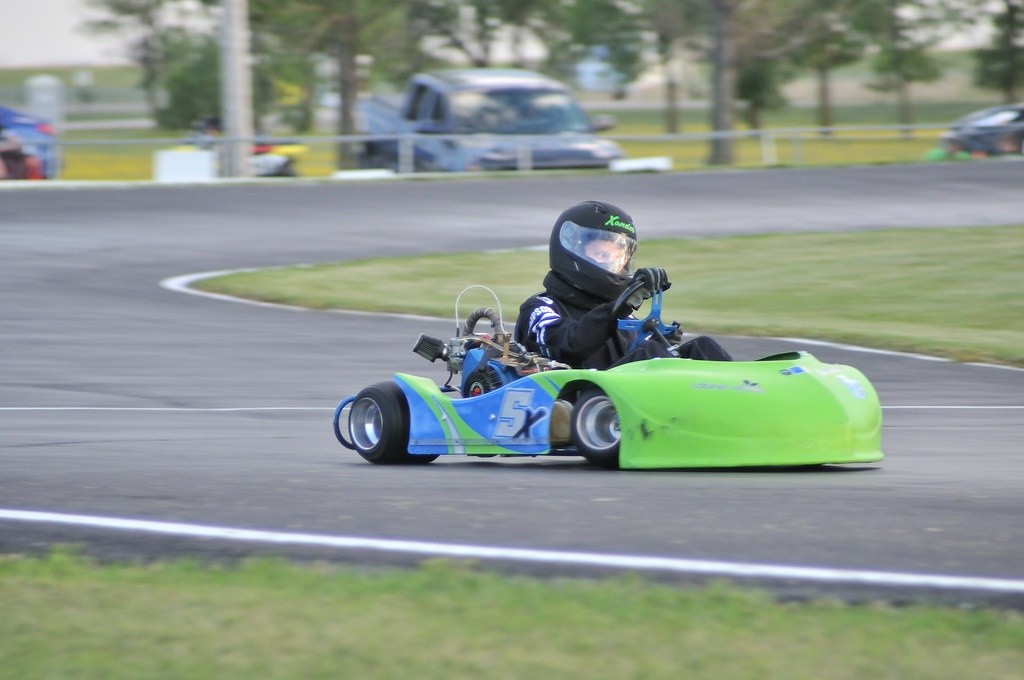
626 267 670 299
668 328 682 345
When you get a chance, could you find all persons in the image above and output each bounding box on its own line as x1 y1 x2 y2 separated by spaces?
0 140 45 179
514 202 734 370
925 137 970 162
202 118 228 177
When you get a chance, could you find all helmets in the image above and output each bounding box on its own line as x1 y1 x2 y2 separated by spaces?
549 200 637 300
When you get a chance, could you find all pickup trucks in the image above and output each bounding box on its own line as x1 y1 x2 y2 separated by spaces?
354 70 625 173
